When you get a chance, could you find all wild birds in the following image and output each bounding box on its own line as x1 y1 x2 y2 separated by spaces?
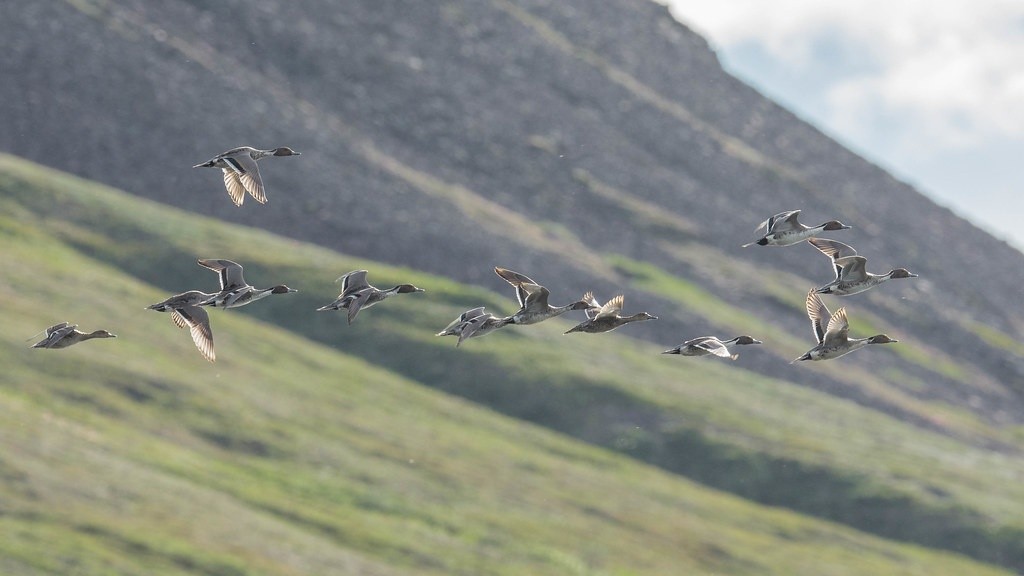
562 291 659 335
788 288 899 365
741 209 853 248
144 291 223 363
434 306 515 348
493 266 598 325
196 259 299 310
316 269 426 328
28 323 118 350
808 237 918 296
660 336 763 361
192 145 303 207
